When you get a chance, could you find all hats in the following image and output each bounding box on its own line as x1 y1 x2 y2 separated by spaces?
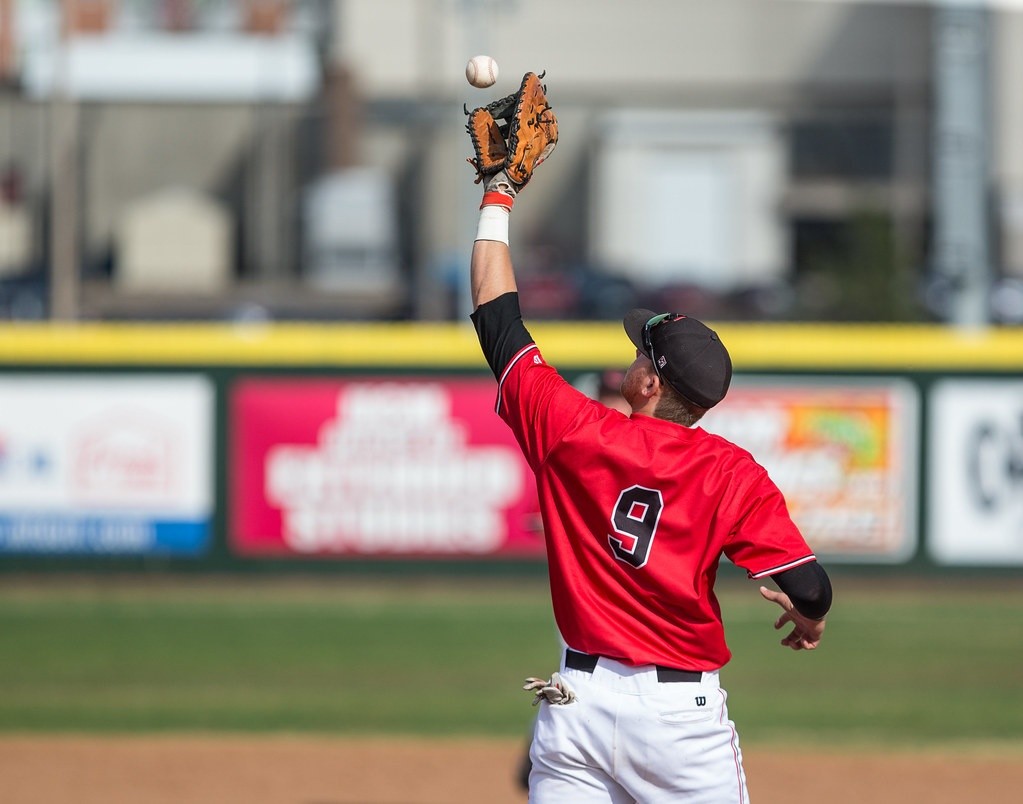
623 308 732 410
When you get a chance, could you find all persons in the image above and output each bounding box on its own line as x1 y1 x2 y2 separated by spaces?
465 72 835 804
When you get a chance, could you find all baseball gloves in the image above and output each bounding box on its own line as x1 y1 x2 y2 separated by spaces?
468 72 558 191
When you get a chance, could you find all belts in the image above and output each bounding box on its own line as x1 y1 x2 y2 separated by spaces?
564 649 703 682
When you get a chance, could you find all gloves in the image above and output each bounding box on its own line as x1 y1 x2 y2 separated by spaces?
480 142 556 212
522 672 576 707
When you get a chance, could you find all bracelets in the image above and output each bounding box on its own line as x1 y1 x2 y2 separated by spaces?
474 207 509 248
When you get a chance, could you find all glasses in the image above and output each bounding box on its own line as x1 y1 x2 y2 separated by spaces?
644 311 689 386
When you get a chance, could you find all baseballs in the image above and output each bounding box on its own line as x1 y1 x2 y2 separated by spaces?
465 54 499 88
